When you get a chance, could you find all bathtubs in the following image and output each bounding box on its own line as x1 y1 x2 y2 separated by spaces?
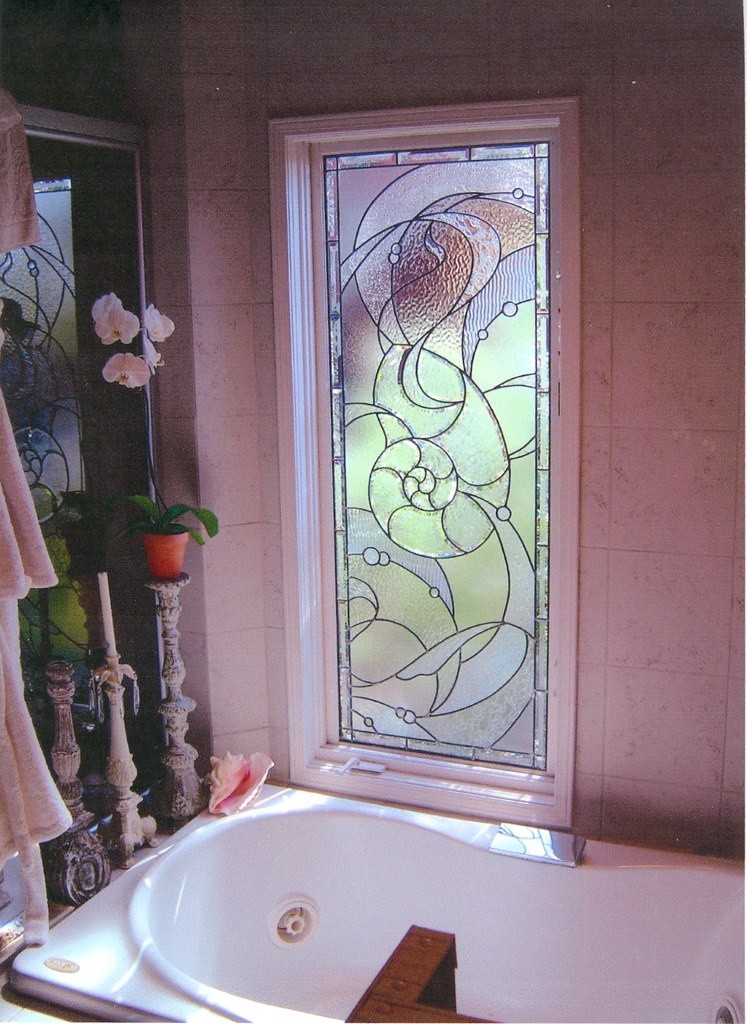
11 785 745 1023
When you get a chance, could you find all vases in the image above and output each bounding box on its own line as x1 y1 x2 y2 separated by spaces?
142 522 190 580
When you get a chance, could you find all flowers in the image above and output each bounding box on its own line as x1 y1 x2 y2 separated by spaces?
92 293 219 545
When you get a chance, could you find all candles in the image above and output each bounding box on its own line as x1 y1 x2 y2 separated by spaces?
98 568 119 658
